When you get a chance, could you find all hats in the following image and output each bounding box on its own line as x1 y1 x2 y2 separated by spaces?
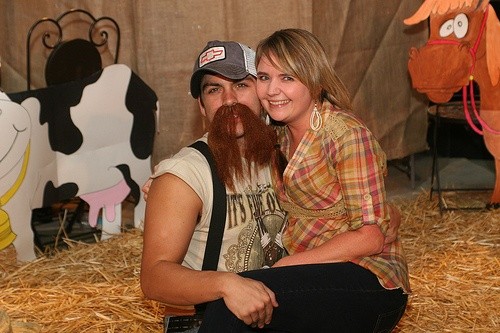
191 40 256 100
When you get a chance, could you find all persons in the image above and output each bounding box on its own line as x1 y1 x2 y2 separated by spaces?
139 38 281 333
142 28 412 333
